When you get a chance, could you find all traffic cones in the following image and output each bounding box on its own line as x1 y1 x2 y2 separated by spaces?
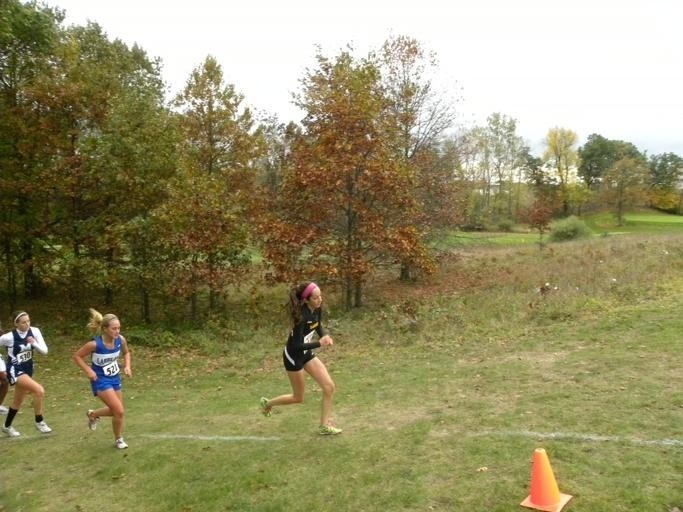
520 447 573 512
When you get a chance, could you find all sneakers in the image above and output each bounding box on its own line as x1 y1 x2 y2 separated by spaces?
115 437 128 448
87 409 100 430
0 406 9 414
261 397 272 417
34 420 52 433
2 424 20 437
319 425 342 434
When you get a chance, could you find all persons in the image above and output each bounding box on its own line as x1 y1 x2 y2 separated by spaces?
73 306 132 450
0 353 9 414
259 279 343 436
0 311 52 438
